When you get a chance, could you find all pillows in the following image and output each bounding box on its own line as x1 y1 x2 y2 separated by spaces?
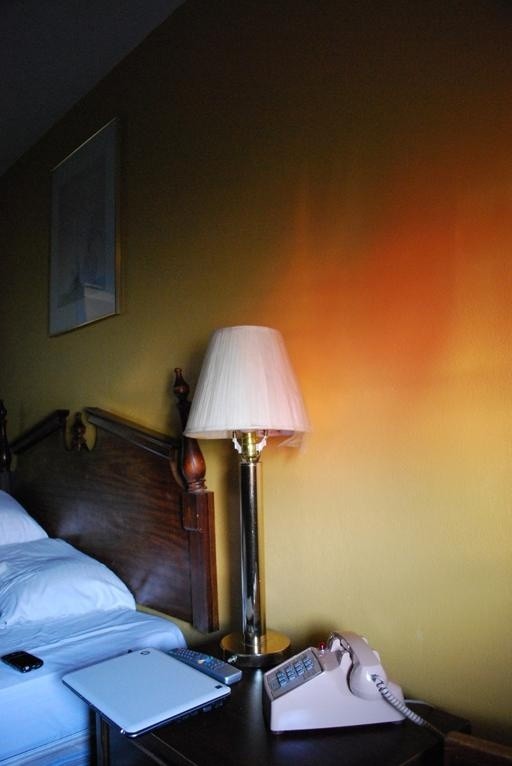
0 483 141 632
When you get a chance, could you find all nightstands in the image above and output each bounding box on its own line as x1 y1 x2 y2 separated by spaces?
89 637 470 766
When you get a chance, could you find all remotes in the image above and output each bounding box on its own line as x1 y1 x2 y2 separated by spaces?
167 647 242 686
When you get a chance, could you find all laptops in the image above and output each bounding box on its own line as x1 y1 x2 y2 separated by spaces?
61 645 233 739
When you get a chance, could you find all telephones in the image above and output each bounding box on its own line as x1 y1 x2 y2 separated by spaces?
262 631 406 736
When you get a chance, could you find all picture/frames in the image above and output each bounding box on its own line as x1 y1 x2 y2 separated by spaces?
46 109 125 341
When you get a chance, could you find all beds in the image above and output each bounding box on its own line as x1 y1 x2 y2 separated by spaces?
0 360 221 766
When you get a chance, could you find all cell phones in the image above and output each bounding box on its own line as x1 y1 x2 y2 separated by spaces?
1 650 44 676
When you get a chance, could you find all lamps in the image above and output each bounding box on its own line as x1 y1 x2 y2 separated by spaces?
182 322 311 671
